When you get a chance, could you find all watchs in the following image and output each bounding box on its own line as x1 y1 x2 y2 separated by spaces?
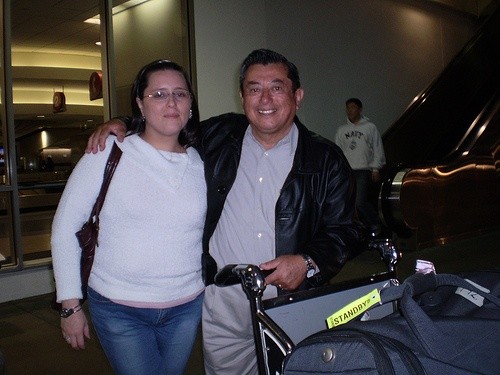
61 304 81 318
302 253 316 278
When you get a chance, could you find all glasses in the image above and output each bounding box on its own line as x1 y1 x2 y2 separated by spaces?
140 88 191 101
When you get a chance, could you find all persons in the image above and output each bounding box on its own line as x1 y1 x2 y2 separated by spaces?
38 156 53 171
50 57 208 375
85 48 367 375
335 98 386 239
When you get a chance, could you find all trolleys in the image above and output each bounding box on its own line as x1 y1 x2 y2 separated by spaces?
213 237 403 375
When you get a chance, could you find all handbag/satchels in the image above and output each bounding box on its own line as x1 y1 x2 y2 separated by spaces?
283 274 500 374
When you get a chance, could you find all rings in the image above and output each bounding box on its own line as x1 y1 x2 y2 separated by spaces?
279 284 282 288
65 338 70 342
